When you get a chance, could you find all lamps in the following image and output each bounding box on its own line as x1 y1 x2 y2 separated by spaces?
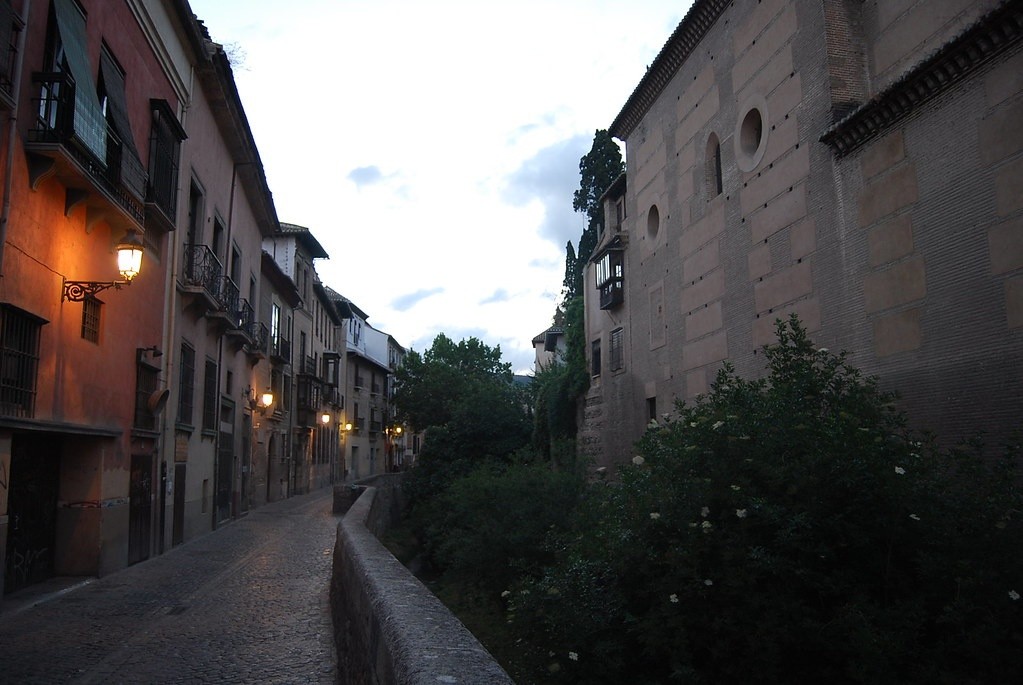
322 410 330 425
346 420 353 431
148 389 171 419
61 228 145 302
138 344 162 357
247 386 273 415
